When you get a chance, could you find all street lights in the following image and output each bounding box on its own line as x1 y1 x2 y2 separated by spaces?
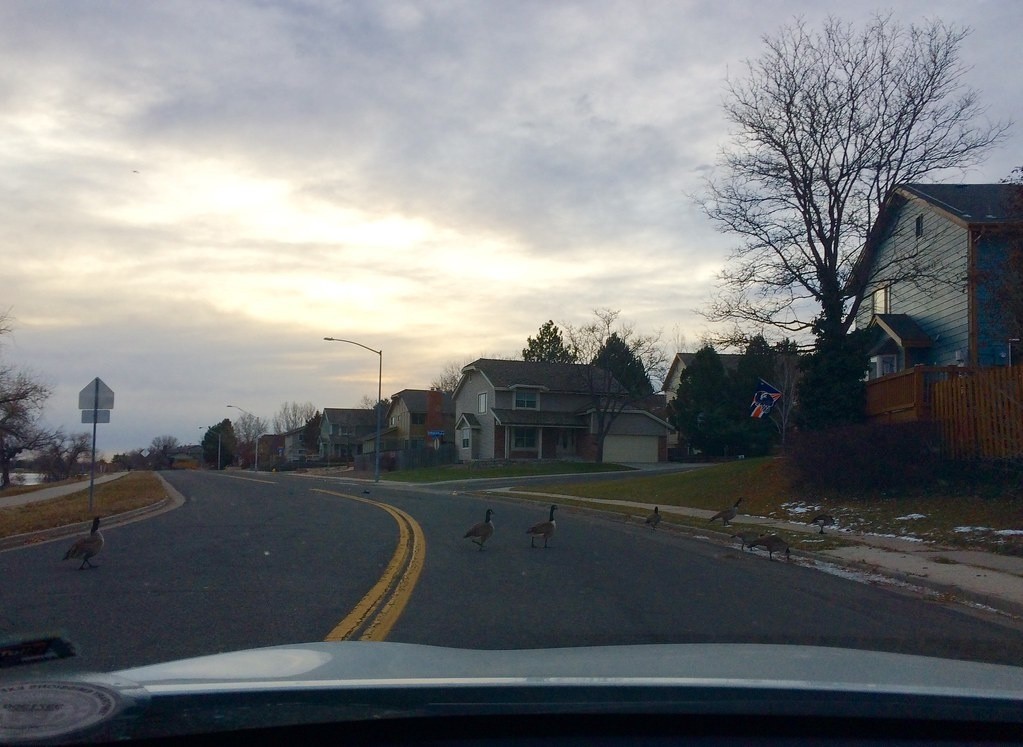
199 427 221 470
227 405 258 471
324 337 382 483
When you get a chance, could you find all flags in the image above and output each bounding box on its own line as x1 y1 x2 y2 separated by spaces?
745 378 783 419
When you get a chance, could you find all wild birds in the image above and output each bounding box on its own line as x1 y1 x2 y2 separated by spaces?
526 504 559 550
61 515 106 570
731 532 791 562
709 496 745 526
646 507 661 530
462 509 495 552
805 514 836 534
